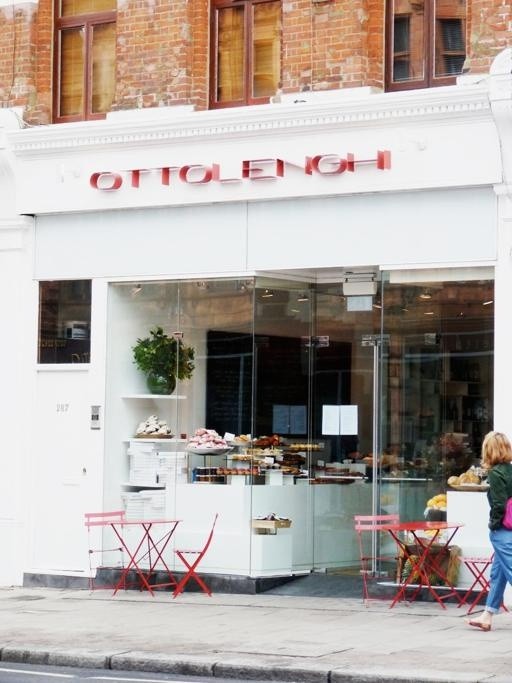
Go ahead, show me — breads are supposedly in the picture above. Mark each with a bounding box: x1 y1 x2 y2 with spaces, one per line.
187 428 229 449
447 468 481 485
280 464 301 475
136 414 171 435
252 435 280 446
235 434 248 442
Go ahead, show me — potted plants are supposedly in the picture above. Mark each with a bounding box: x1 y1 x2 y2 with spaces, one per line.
133 325 197 395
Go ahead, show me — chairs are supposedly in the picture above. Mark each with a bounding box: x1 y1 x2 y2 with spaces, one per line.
355 513 507 612
80 508 221 598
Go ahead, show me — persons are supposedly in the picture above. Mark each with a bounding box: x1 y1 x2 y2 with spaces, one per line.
465 430 512 632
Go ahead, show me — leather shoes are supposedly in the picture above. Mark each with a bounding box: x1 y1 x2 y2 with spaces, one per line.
464 618 491 631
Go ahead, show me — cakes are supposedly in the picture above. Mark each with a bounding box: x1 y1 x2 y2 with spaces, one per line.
288 443 320 451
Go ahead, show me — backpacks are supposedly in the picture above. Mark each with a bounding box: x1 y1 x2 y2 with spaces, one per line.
502 496 512 529
